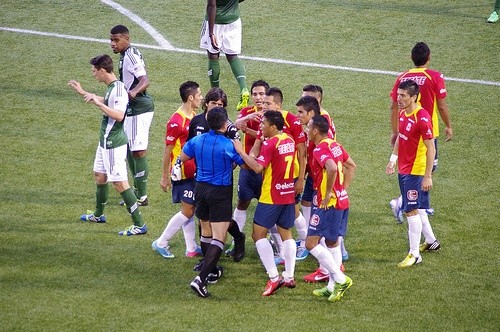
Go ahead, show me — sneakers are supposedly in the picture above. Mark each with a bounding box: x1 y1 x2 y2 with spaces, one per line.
233 233 245 262
420 240 441 252
304 268 329 283
80 212 107 223
313 276 353 303
262 277 296 296
193 259 204 271
397 252 422 267
151 241 174 259
119 223 148 235
208 266 223 283
237 88 250 111
119 195 148 206
189 275 210 298
186 246 203 257
225 244 233 256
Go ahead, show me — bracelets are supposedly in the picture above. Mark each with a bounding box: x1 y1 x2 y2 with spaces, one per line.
390 154 398 162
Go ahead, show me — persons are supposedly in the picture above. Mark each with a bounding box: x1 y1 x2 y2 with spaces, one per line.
231 110 299 296
303 116 353 302
105 25 154 207
293 85 349 261
180 106 262 298
294 95 346 282
487 0 500 23
149 81 205 259
202 0 250 110
225 79 284 257
385 80 442 267
67 54 147 236
386 41 453 223
186 86 246 270
240 88 310 265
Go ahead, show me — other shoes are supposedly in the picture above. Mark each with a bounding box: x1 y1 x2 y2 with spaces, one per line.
296 247 309 260
342 250 349 261
389 198 404 224
486 11 499 22
426 208 435 215
274 256 284 264
